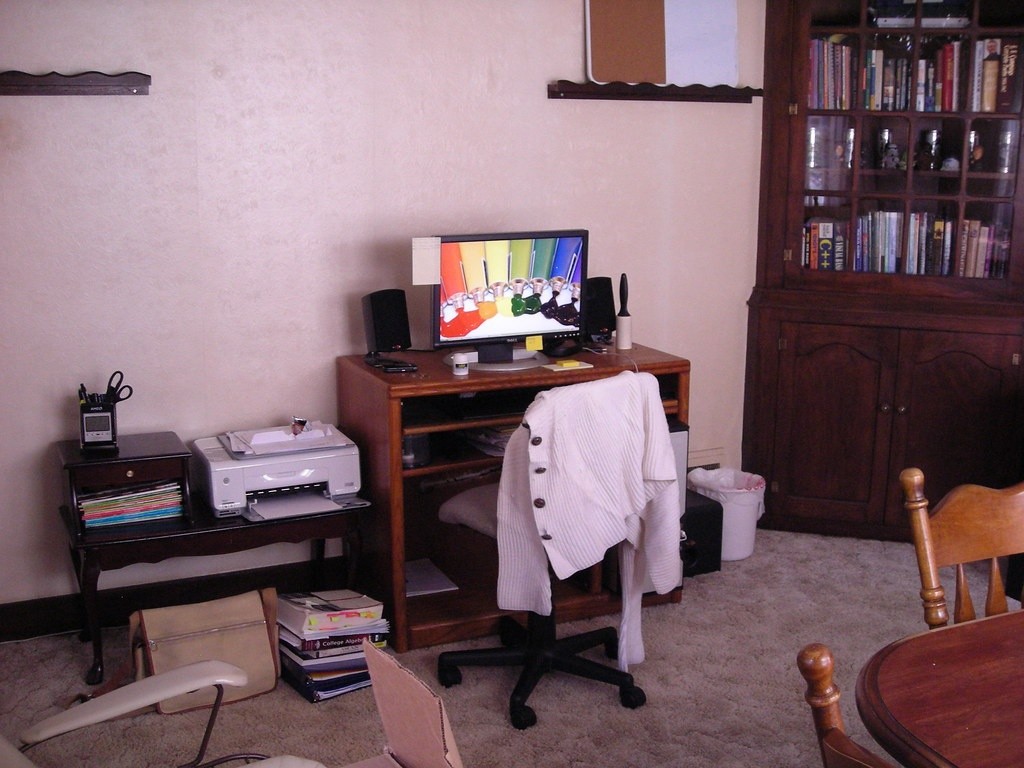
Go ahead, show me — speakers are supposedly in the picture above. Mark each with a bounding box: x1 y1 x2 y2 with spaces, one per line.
585 276 616 349
360 288 412 367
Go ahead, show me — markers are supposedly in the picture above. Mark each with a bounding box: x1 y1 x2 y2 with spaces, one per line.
78 383 90 405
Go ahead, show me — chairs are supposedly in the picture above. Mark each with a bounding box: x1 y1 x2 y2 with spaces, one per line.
432 371 678 729
798 638 911 768
899 467 1024 624
0 658 327 768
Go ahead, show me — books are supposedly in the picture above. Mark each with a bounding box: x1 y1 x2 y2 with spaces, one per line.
279 619 390 703
802 211 1008 278
75 480 183 527
808 38 1018 114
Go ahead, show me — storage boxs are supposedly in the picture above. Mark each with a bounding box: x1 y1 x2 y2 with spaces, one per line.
277 590 382 634
341 635 466 768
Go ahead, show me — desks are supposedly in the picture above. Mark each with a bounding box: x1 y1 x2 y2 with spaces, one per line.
335 340 700 652
68 501 387 686
854 610 1023 767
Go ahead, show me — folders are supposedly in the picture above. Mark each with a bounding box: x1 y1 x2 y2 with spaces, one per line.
280 651 373 703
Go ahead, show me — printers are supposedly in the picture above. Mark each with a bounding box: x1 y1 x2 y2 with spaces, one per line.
193 422 371 522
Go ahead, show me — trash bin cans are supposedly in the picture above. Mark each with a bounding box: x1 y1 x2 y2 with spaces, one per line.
688 468 765 562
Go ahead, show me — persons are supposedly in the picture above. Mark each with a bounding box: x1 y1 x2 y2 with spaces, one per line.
984 41 999 60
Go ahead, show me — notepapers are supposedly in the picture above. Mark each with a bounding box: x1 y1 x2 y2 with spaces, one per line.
556 359 579 369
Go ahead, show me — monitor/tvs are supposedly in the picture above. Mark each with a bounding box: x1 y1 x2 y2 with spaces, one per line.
430 229 588 370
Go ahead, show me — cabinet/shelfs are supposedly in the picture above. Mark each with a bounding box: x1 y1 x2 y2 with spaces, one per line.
734 0 1024 540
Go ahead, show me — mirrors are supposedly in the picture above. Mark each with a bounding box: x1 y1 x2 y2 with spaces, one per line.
584 0 739 88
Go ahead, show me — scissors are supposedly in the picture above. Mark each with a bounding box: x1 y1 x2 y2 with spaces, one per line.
105 371 134 404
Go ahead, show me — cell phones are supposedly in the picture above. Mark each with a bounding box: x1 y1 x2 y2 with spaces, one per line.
384 364 418 373
